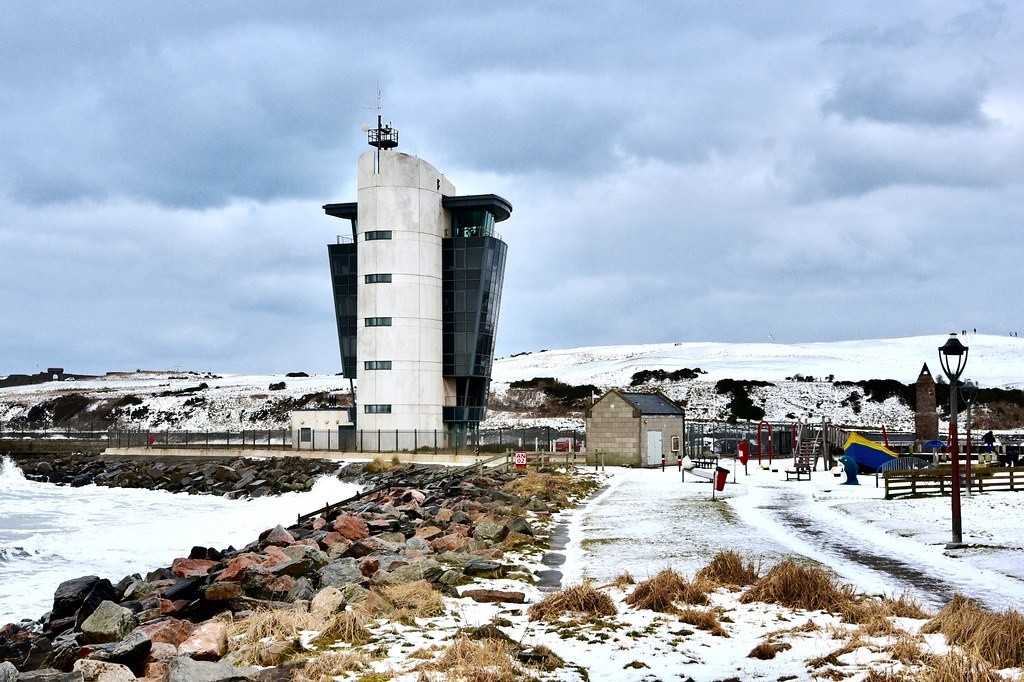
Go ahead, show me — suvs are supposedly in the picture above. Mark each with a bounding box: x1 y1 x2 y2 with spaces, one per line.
717 439 757 455
685 437 722 453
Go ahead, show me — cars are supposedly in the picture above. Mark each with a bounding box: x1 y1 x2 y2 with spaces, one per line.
551 438 581 452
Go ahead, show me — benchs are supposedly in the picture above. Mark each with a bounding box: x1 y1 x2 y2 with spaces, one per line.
875 456 940 486
691 456 718 468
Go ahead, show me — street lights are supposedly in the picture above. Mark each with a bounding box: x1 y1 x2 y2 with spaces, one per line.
937 333 969 541
